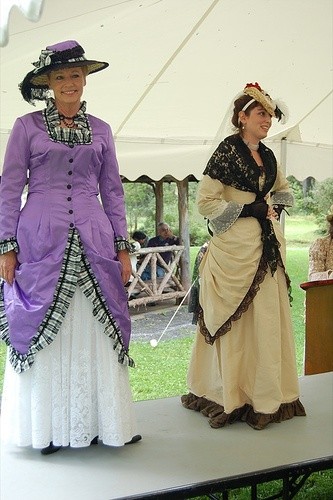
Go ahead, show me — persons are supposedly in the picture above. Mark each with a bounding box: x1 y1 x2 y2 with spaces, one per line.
0 40 142 454
181 83 306 431
308 204 333 281
136 223 179 281
129 231 150 249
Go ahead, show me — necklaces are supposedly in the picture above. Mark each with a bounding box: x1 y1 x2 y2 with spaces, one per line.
59 115 75 128
242 137 259 151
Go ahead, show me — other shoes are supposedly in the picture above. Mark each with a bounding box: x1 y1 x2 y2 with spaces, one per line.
40 441 60 455
124 434 141 444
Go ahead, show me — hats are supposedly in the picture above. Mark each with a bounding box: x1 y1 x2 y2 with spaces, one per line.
243 82 288 125
18 40 109 106
